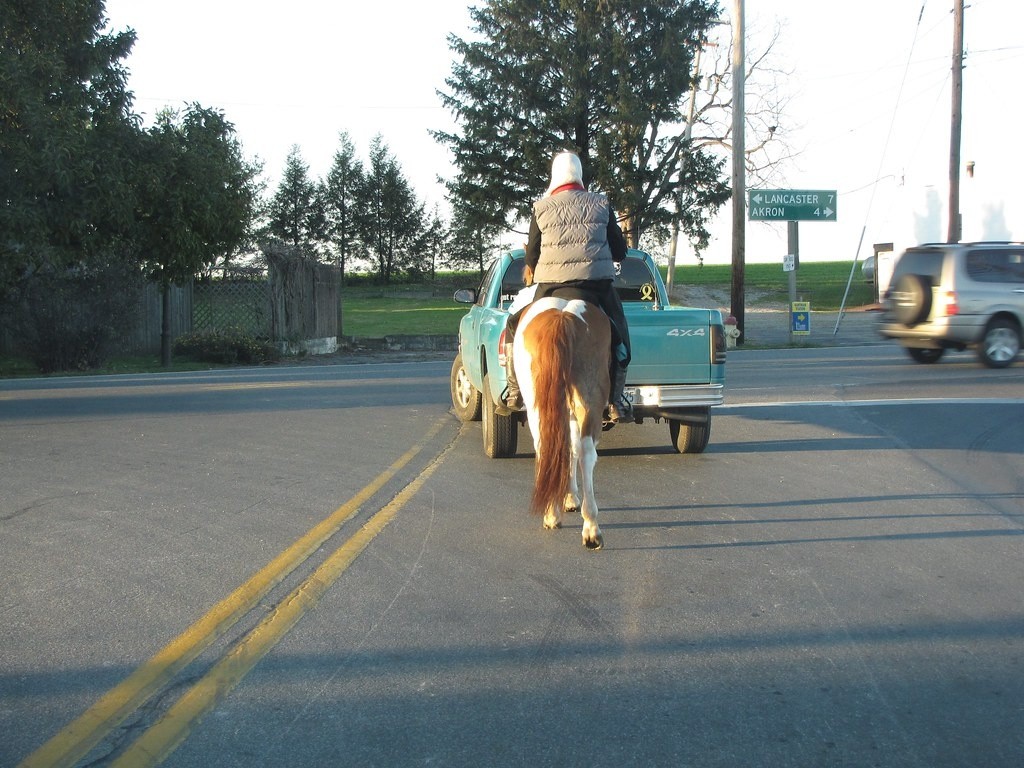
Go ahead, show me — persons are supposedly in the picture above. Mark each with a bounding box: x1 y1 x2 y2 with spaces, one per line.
506 153 634 423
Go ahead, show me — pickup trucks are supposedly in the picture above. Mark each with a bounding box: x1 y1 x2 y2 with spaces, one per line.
450 249 727 459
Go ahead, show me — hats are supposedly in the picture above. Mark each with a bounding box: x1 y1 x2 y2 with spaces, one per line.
542 148 585 197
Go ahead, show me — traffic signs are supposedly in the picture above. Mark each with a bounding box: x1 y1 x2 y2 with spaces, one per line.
792 302 811 336
748 189 837 222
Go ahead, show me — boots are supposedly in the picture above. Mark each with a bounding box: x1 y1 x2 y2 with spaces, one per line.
495 343 523 417
602 368 626 431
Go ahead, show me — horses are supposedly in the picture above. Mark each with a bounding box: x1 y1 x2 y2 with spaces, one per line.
510 296 613 552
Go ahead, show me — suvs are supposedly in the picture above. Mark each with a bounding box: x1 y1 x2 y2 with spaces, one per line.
875 243 1023 370
